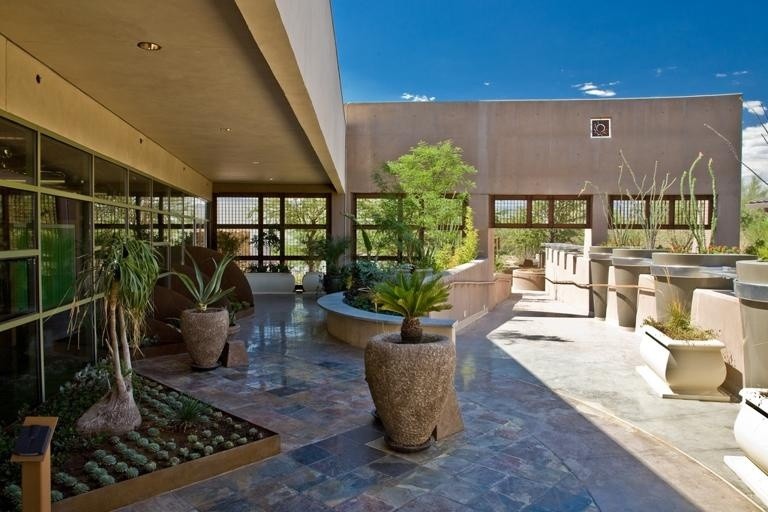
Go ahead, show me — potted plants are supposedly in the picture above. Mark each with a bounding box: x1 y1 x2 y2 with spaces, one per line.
365 267 455 453
248 262 288 273
635 264 731 403
169 248 239 371
298 230 364 292
